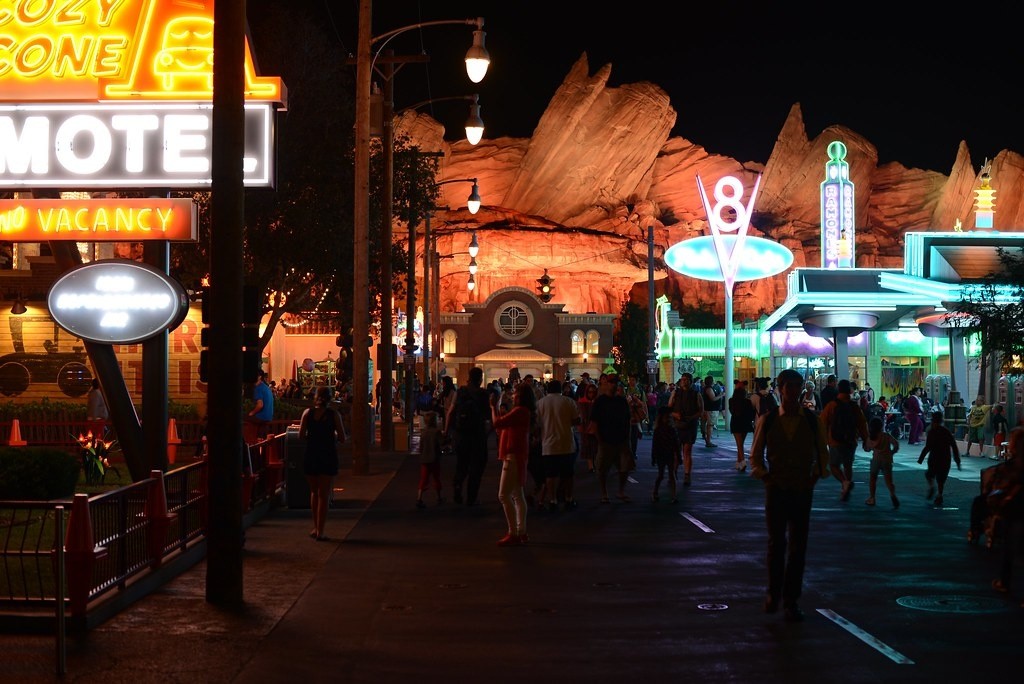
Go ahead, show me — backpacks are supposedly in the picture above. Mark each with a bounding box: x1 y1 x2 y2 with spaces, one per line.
830 407 861 447
758 393 777 417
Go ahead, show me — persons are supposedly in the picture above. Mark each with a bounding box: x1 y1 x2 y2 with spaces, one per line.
372 375 418 421
647 365 726 500
418 359 645 548
295 387 349 544
239 368 275 424
728 360 898 627
277 377 348 401
86 377 109 423
900 384 1024 624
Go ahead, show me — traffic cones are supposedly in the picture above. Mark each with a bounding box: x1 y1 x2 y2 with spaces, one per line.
8 418 28 448
167 418 185 445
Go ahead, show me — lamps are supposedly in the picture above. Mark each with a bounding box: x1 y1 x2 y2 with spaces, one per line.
464 94 485 145
469 257 477 275
468 178 481 214
468 274 475 290
464 17 491 83
469 230 479 257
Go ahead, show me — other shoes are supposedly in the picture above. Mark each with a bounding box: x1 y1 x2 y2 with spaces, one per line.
908 438 927 445
498 532 531 548
897 433 904 441
961 453 1008 460
928 487 944 502
766 591 806 623
407 464 693 507
704 440 901 508
309 530 330 542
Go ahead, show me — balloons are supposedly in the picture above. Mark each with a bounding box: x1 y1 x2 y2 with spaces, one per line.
302 358 315 371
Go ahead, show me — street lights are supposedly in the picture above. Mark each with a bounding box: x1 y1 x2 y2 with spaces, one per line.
354 2 492 477
